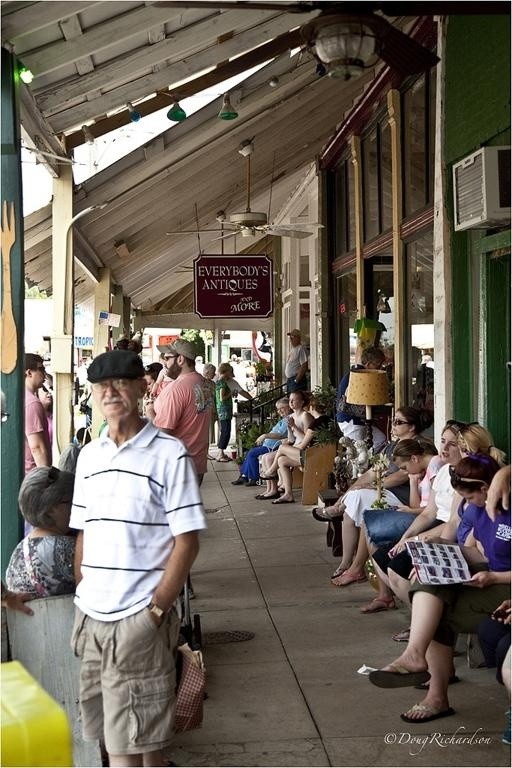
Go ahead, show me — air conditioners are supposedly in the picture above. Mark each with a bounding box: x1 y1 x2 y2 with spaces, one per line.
451 145 510 235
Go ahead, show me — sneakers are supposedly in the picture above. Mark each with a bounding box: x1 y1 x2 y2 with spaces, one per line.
207 454 233 462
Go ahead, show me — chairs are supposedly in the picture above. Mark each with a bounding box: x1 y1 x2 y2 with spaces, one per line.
5 594 110 767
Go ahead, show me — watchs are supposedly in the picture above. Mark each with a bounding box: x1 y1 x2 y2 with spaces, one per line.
147 602 167 621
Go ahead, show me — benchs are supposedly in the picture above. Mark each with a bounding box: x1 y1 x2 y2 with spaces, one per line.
294 440 340 505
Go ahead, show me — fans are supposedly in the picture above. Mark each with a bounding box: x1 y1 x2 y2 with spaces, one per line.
173 211 227 273
164 147 325 242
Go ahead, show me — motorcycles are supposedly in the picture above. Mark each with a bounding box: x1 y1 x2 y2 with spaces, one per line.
243 361 257 392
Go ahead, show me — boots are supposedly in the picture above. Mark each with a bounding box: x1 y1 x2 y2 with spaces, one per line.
231 476 258 487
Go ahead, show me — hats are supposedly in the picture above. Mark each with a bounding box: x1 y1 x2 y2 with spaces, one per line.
287 328 302 336
156 339 196 360
87 350 145 383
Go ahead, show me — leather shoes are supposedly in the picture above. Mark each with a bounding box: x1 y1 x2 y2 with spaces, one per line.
259 471 279 481
255 492 280 500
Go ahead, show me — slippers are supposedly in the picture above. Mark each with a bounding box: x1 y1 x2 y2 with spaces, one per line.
370 663 431 688
400 703 455 723
272 498 295 504
313 507 343 522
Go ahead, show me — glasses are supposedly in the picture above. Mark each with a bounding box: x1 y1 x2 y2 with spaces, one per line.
44 466 61 489
42 385 53 392
92 379 130 391
163 355 177 361
35 367 45 372
395 421 409 425
447 465 489 490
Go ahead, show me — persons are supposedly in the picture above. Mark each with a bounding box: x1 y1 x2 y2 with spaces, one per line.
2 580 37 616
5 463 81 600
19 347 226 534
145 337 213 493
67 347 211 765
226 327 511 753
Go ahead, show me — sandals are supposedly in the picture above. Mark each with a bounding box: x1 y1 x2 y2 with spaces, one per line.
332 571 368 587
360 598 399 615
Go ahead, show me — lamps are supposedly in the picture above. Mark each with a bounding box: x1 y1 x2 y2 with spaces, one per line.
314 0 378 80
345 367 395 465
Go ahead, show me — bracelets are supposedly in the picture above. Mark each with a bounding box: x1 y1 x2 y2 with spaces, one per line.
145 400 154 406
1 588 13 601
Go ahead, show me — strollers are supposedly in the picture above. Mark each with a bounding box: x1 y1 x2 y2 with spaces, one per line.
173 571 205 648
